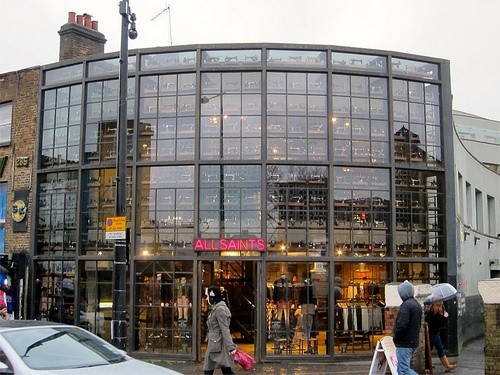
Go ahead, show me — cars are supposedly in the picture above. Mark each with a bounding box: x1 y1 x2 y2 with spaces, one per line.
0 319 185 375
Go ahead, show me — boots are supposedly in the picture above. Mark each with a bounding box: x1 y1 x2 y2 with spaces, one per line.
425 369 431 375
440 356 457 372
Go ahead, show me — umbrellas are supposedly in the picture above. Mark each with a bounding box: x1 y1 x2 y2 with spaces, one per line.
423 283 460 312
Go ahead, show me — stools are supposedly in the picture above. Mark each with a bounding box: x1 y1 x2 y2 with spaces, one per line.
274 338 293 355
299 337 318 355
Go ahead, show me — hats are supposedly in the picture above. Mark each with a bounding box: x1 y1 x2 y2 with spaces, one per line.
206 286 222 305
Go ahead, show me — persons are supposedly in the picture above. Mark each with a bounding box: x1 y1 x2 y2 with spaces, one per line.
272 273 293 339
334 277 343 303
393 281 422 375
152 275 163 323
174 277 192 326
424 302 457 372
202 286 237 375
0 306 11 320
297 279 317 340
220 286 230 309
